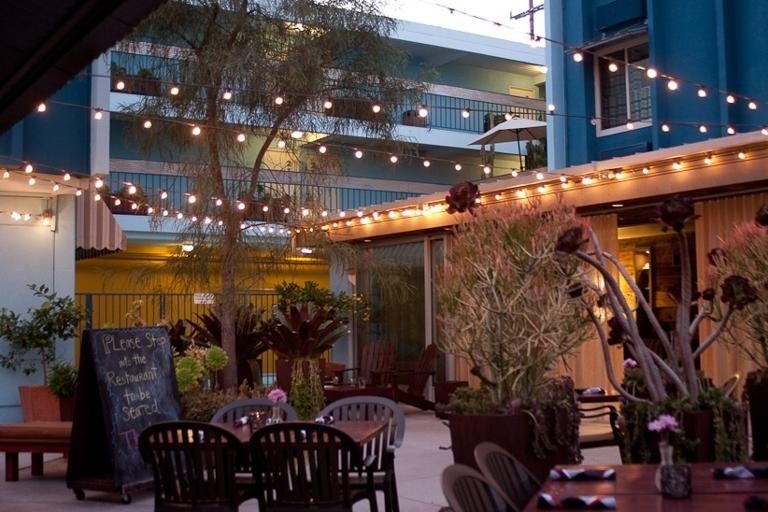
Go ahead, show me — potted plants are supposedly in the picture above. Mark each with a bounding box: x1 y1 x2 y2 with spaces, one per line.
0 282 90 422
438 193 586 472
182 300 350 396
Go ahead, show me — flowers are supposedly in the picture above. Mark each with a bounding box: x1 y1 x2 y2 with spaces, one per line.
648 413 679 443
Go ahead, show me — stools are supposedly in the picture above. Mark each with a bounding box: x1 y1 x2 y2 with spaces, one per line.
0 420 77 481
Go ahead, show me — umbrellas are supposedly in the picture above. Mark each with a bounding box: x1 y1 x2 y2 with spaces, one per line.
467 115 547 173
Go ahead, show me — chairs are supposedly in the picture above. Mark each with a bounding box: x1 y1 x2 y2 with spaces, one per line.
335 334 437 411
440 443 539 512
136 395 407 512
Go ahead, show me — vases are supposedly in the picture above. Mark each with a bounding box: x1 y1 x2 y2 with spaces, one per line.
654 442 675 493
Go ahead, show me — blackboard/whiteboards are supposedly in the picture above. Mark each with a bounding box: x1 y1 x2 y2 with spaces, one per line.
90 325 187 488
